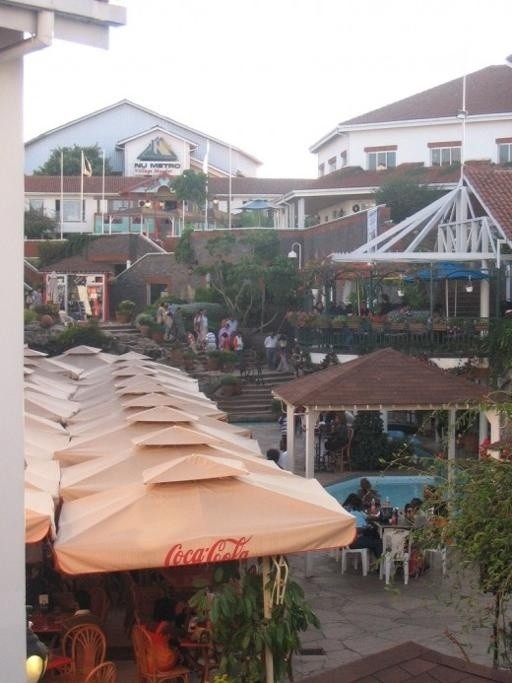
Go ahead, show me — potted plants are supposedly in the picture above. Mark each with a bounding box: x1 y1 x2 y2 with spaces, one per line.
116 300 241 395
290 310 491 332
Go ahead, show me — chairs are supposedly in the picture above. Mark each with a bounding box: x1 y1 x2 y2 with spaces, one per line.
341 528 447 584
43 585 190 683
331 427 354 473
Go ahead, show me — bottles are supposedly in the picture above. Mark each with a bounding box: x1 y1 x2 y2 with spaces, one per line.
392 508 398 525
370 497 376 514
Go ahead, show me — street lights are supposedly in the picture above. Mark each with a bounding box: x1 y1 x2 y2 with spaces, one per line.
288 242 302 269
456 109 468 186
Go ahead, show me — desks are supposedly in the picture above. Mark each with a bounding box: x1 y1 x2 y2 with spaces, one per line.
373 517 416 543
28 611 63 647
179 637 229 683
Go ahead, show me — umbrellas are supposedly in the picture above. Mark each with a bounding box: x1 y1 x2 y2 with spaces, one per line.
442 268 488 318
404 263 462 320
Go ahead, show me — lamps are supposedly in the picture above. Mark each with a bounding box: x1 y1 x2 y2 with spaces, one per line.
26 653 49 683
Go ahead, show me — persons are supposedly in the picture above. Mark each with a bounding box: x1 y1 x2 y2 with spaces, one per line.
337 474 449 579
146 597 206 679
265 446 281 468
278 435 288 469
159 301 290 370
358 293 400 319
325 413 350 463
